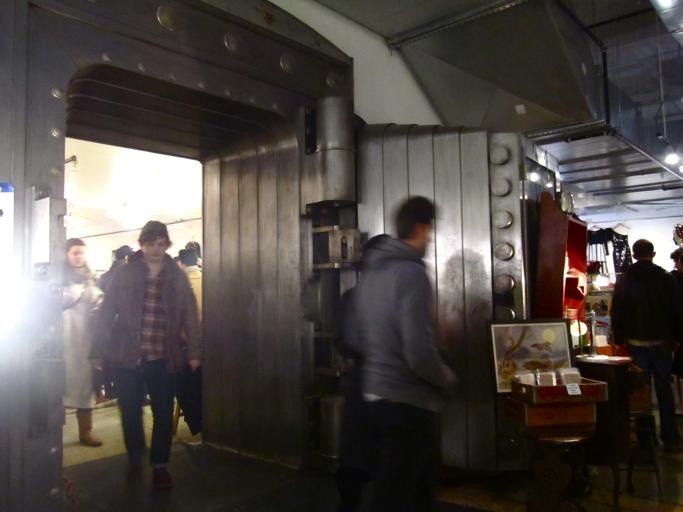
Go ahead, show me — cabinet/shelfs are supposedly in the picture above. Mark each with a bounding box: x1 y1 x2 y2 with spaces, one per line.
536 191 589 322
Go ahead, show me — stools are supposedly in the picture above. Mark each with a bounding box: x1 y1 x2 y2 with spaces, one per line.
521 426 596 512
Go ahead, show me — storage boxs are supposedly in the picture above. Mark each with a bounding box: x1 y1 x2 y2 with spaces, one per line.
502 377 609 429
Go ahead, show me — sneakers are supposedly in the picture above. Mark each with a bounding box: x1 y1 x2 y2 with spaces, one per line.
185 434 202 445
80 427 102 446
154 468 171 489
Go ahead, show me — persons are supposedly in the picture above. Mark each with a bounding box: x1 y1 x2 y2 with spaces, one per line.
63 220 202 487
670 247 683 379
609 240 683 454
334 234 391 512
346 196 457 512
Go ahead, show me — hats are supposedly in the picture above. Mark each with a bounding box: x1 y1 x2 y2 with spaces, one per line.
141 221 167 234
